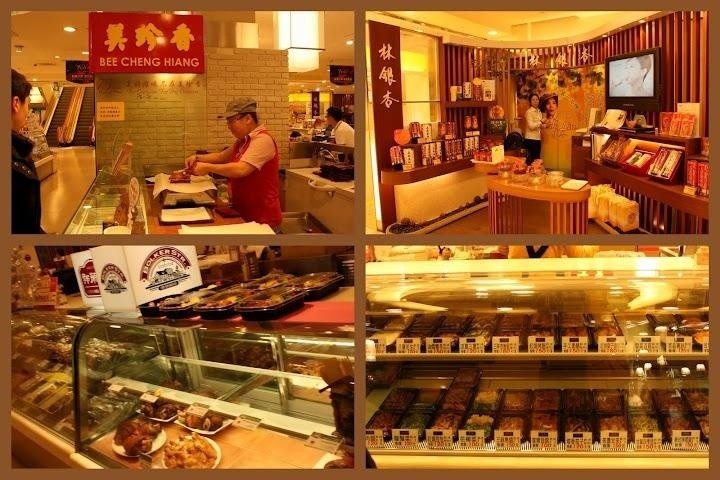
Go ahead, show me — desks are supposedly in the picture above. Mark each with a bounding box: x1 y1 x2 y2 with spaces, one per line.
484 169 587 233
65 179 256 235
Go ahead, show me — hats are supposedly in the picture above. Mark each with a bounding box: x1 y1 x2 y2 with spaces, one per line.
216 97 257 119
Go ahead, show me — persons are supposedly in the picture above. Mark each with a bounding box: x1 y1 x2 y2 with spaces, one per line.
539 92 559 119
11 68 48 234
326 106 354 148
183 96 283 236
610 55 652 97
524 93 548 164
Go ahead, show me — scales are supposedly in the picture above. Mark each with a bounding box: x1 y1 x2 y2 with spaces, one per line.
159 190 216 209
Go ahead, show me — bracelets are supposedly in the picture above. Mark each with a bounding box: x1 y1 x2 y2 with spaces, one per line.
195 155 197 161
540 120 544 124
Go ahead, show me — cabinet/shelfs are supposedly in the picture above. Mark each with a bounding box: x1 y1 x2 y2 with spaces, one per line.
12 305 355 468
362 247 714 470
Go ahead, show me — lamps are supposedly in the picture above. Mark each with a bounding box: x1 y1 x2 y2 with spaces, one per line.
253 8 327 73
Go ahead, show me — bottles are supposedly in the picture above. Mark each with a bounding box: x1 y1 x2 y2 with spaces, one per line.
496 159 564 188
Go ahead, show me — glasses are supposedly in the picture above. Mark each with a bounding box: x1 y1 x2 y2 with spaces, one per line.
227 113 248 124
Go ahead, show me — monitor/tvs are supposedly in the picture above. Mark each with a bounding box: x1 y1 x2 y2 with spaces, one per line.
605 47 661 112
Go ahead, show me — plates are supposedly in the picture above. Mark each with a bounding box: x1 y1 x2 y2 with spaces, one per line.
111 407 235 469
